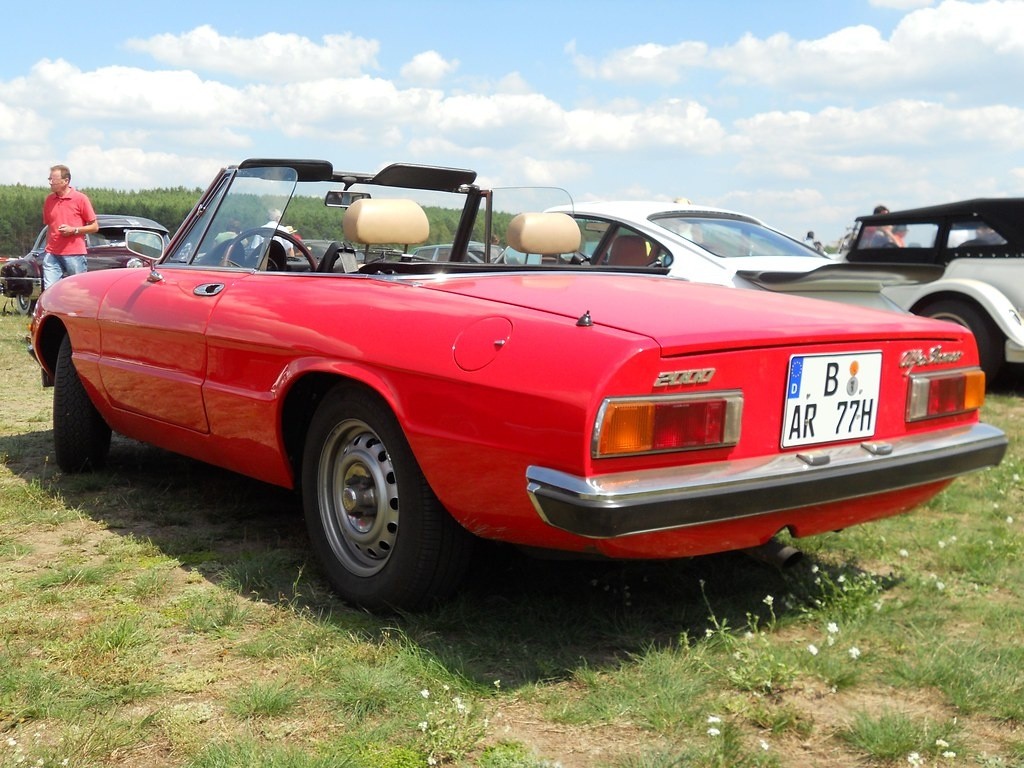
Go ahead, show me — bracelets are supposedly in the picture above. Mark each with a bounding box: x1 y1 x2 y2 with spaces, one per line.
75 227 79 235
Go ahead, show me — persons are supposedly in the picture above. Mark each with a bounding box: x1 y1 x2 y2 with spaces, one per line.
42 165 99 291
491 234 499 245
859 205 1007 247
804 231 828 257
246 209 295 258
284 226 304 257
212 220 249 248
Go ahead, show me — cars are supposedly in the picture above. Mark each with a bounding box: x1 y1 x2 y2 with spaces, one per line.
27 158 1009 618
0 215 172 316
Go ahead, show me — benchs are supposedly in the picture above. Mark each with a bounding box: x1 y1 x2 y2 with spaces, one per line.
344 188 672 278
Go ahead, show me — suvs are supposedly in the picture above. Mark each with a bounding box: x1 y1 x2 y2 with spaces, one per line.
825 198 1023 391
